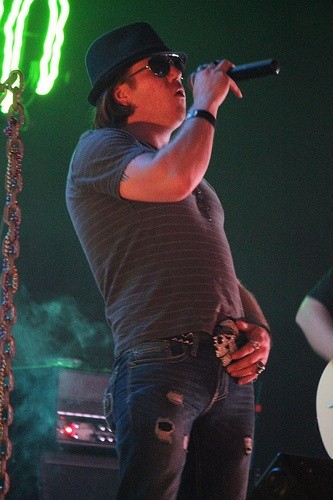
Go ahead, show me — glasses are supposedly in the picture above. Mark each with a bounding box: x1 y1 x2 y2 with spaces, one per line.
127 52 187 78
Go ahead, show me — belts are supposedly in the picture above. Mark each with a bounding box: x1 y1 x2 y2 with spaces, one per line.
161 316 249 367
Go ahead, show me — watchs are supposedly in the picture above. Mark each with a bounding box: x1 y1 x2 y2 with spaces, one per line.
187 109 217 126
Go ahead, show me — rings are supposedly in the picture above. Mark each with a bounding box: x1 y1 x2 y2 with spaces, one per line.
249 340 261 350
255 361 265 377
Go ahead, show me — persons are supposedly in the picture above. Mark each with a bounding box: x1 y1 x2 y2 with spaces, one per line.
295 265 333 363
65 21 272 500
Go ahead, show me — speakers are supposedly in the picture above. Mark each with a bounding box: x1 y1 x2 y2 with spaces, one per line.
247 452 333 500
39 450 120 500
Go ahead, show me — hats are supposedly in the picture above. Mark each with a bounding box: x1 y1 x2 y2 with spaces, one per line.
84 22 187 107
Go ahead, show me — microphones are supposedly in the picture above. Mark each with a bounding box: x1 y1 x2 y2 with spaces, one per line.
187 58 280 93
